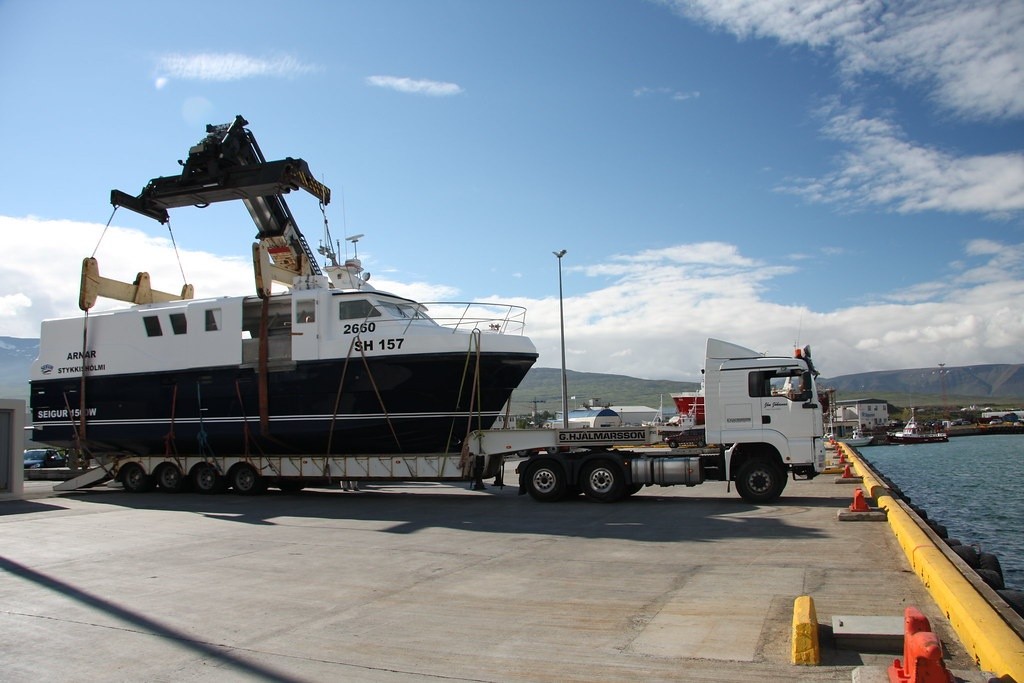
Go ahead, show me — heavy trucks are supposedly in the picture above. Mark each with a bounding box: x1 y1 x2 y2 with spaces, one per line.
23 286 841 501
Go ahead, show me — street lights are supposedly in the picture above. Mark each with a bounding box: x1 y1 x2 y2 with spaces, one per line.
553 249 569 429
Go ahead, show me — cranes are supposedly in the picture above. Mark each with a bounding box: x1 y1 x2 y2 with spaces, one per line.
108 115 332 285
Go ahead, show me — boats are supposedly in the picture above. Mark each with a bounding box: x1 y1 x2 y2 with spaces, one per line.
889 407 951 444
26 287 539 454
